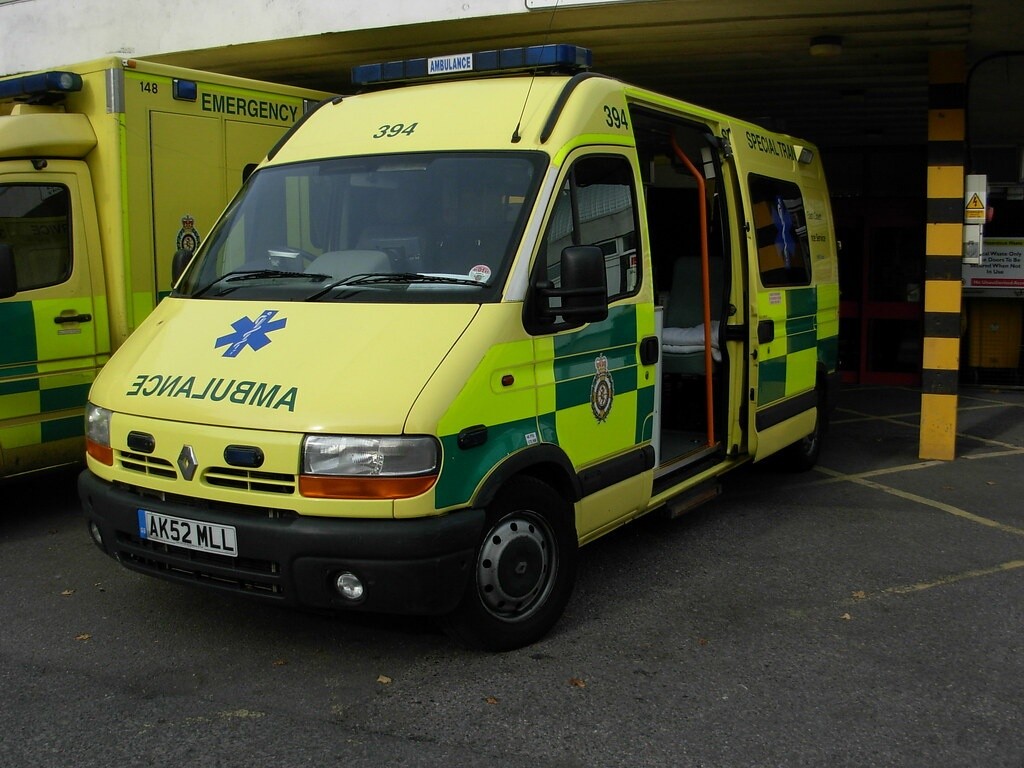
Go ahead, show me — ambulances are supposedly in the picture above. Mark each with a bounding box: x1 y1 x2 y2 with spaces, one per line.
77 40 848 652
0 56 364 485
